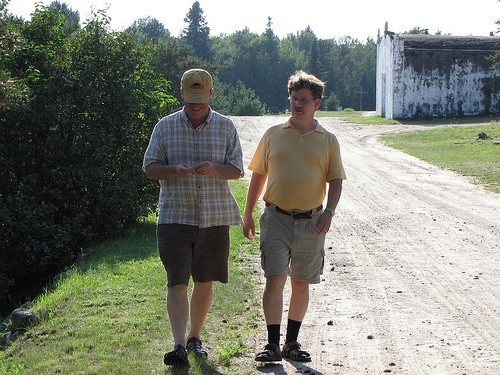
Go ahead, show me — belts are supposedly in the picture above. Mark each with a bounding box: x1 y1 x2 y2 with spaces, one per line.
265 201 323 220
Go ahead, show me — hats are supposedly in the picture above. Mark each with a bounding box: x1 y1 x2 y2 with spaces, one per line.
181 69 212 103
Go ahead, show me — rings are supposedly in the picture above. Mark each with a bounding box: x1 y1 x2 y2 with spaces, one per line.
324 229 327 232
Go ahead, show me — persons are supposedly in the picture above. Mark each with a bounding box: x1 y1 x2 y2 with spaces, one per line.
141 68 244 366
242 70 347 361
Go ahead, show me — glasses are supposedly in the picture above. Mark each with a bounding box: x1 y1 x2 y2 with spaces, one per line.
291 96 315 104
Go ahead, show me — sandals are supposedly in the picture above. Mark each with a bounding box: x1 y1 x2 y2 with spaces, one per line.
282 342 311 362
164 346 189 366
254 343 282 362
187 337 208 359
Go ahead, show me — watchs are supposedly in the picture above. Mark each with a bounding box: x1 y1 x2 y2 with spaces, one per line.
325 207 335 216
